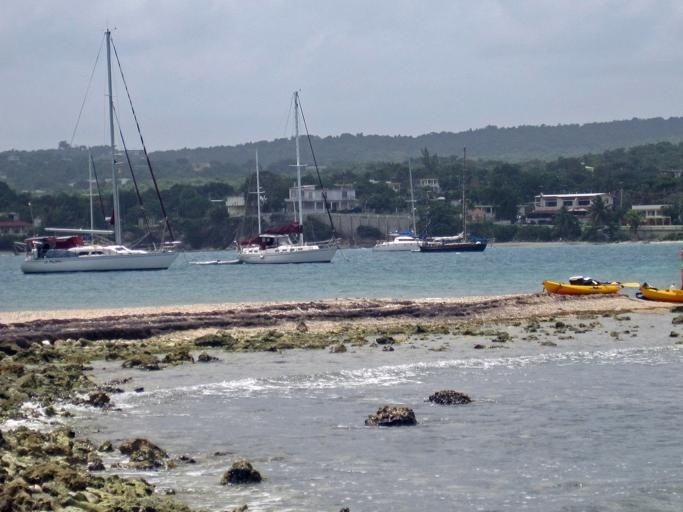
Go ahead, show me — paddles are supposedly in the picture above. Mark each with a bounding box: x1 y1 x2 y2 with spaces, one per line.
601 282 640 287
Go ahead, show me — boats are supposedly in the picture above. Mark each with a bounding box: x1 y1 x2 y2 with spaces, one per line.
640 283 683 302
374 147 490 251
544 280 622 294
234 91 342 264
21 30 181 273
